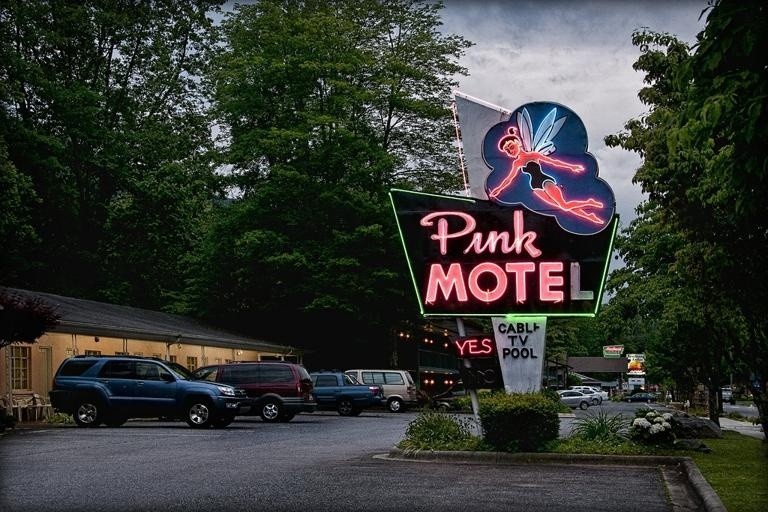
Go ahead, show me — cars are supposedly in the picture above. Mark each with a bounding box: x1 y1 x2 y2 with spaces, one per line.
592 387 600 391
557 389 592 411
297 373 386 417
666 391 673 399
574 389 602 406
624 393 657 403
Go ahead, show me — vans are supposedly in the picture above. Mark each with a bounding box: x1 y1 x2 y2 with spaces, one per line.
188 359 316 421
343 369 417 415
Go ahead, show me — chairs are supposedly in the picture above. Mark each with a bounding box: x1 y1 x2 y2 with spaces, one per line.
1 394 30 423
33 394 53 420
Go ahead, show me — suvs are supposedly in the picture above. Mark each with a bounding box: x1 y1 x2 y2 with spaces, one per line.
432 381 472 411
720 388 736 405
49 353 242 427
75 353 249 427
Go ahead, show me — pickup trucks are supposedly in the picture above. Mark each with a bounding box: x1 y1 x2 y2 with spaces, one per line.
572 386 609 405
